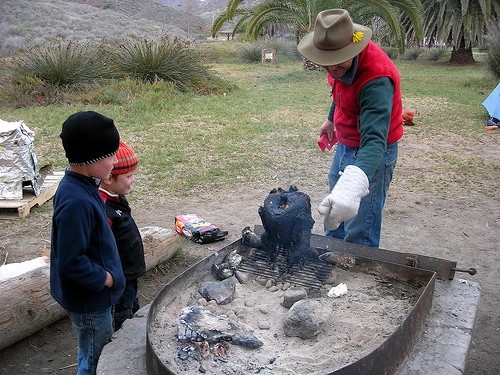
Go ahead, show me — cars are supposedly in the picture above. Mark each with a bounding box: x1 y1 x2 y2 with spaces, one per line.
420 37 446 48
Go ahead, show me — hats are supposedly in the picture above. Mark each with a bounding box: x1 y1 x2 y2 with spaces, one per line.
111 140 138 174
297 9 372 66
59 111 119 165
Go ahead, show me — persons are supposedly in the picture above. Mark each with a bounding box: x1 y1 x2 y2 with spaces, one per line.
294 7 405 250
429 35 435 49
47 110 127 375
96 139 148 331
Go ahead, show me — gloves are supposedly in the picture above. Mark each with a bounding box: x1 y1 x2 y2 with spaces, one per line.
318 165 370 230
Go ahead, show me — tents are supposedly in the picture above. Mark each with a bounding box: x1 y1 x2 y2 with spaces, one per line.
481 82 500 128
